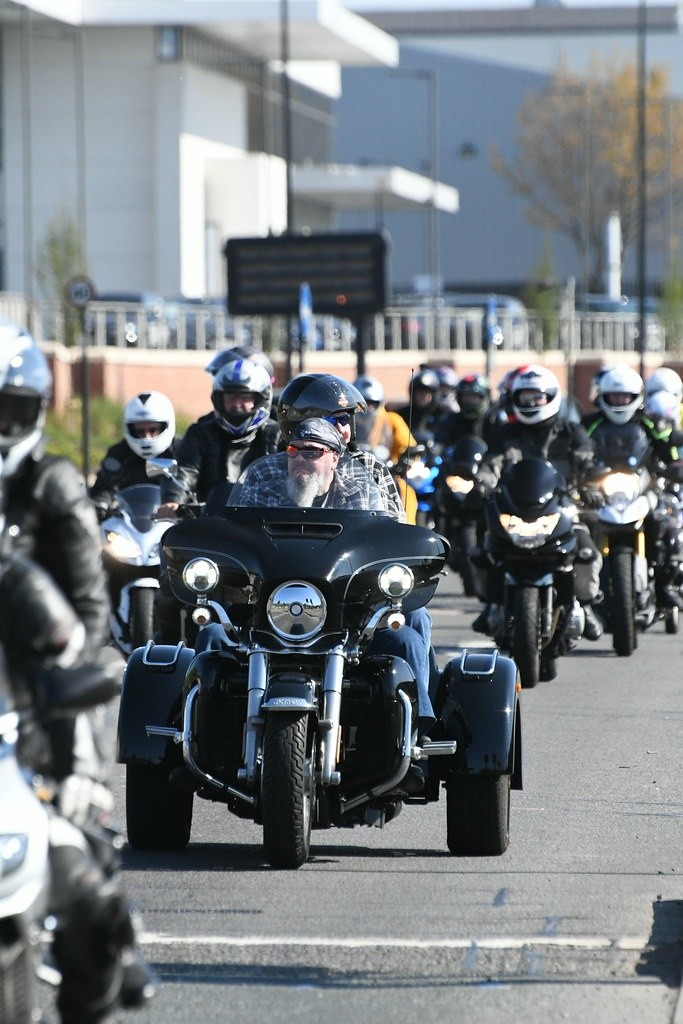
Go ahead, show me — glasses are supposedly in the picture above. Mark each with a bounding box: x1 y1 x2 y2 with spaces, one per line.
286 443 333 460
324 414 351 428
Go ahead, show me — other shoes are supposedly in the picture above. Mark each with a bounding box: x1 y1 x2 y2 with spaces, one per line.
471 604 490 635
49 813 159 1024
579 601 603 639
659 581 683 608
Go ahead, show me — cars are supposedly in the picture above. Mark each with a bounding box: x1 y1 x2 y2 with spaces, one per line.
81 291 356 350
368 295 664 349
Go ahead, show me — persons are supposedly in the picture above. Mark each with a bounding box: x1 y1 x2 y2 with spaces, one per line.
464 365 606 640
349 364 492 529
0 333 162 1024
165 417 438 795
581 365 682 610
92 388 185 523
273 374 434 742
153 346 287 526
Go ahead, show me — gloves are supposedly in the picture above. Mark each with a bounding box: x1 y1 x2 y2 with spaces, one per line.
580 479 612 511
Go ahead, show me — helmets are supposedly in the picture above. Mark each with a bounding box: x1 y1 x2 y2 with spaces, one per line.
455 373 490 419
1 321 53 476
123 390 176 459
204 345 274 377
435 366 457 407
409 370 441 413
509 363 561 424
496 371 511 403
644 366 682 431
275 373 368 444
597 367 645 424
210 360 273 437
352 375 383 402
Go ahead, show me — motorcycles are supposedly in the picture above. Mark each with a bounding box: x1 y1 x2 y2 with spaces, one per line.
114 507 524 870
457 435 612 689
346 408 683 656
0 664 120 1023
99 484 182 660
